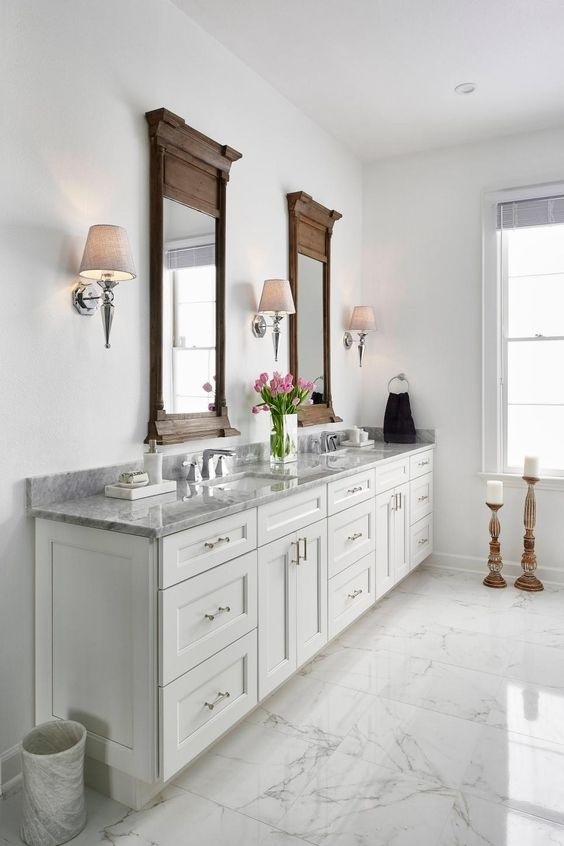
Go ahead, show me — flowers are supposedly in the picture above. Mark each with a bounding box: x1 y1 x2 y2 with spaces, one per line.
201 375 215 410
249 370 316 457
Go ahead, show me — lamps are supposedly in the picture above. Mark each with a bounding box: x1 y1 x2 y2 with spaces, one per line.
343 304 377 367
71 224 137 350
252 280 296 360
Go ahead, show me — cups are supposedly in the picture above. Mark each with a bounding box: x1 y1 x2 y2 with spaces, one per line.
335 431 346 445
309 439 322 454
360 432 369 442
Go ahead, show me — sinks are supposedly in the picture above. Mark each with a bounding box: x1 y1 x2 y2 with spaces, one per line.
320 447 394 457
194 470 298 494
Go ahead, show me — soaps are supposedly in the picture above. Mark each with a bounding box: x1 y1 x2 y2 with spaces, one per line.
121 471 147 483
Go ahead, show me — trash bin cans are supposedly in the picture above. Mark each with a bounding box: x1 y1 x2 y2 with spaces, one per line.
19 719 88 846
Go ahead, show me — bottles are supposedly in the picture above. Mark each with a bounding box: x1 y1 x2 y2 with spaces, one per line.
348 425 360 443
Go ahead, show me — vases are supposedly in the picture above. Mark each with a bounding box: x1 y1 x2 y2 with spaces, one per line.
269 413 299 462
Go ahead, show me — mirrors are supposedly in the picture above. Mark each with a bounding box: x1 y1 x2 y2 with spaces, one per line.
144 108 241 445
286 189 344 429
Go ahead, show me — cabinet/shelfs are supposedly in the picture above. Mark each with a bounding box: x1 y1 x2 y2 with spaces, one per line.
159 506 260 782
409 449 435 572
376 456 411 600
326 467 377 643
258 483 329 701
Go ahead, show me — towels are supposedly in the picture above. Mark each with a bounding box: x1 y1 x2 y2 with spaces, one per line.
382 392 417 443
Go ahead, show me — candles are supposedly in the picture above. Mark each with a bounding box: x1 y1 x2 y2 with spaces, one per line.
486 480 503 504
523 454 540 477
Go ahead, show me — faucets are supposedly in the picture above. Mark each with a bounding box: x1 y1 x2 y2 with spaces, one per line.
320 431 337 453
202 448 236 478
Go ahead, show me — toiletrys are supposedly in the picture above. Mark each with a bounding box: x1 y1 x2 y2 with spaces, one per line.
350 425 360 442
143 439 164 483
